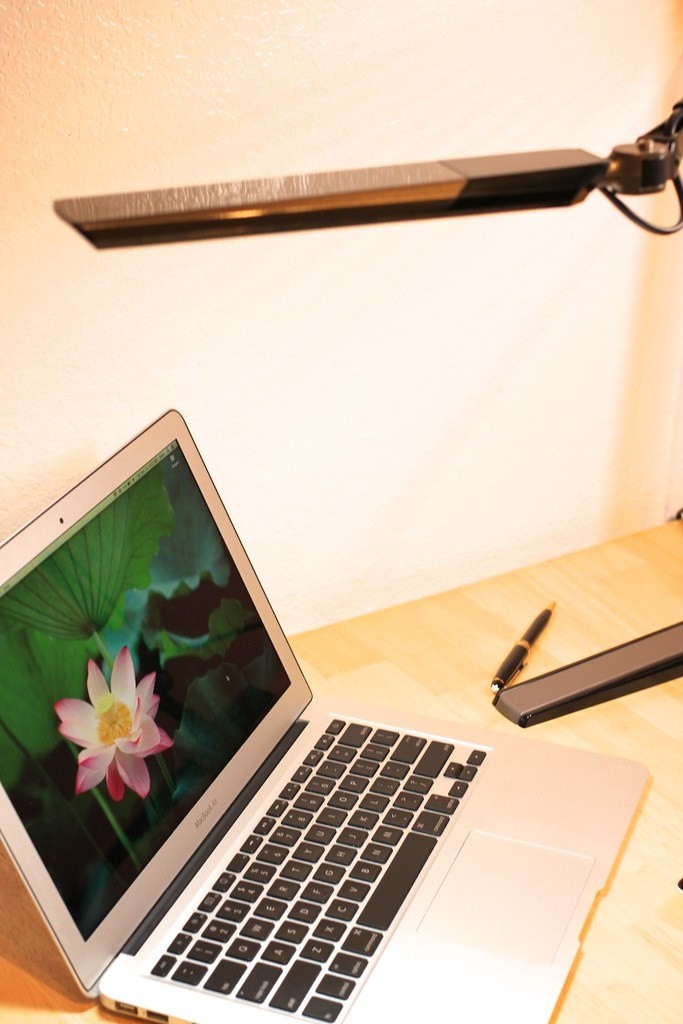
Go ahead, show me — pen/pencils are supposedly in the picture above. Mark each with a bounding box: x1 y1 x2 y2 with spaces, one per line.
489 599 559 694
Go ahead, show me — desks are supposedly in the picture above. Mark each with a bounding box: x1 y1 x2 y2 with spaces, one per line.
0 518 682 1024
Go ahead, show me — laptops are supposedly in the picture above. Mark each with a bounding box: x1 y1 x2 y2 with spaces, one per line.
0 407 648 1024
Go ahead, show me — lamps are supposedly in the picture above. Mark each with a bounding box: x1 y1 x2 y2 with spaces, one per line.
50 92 682 729
